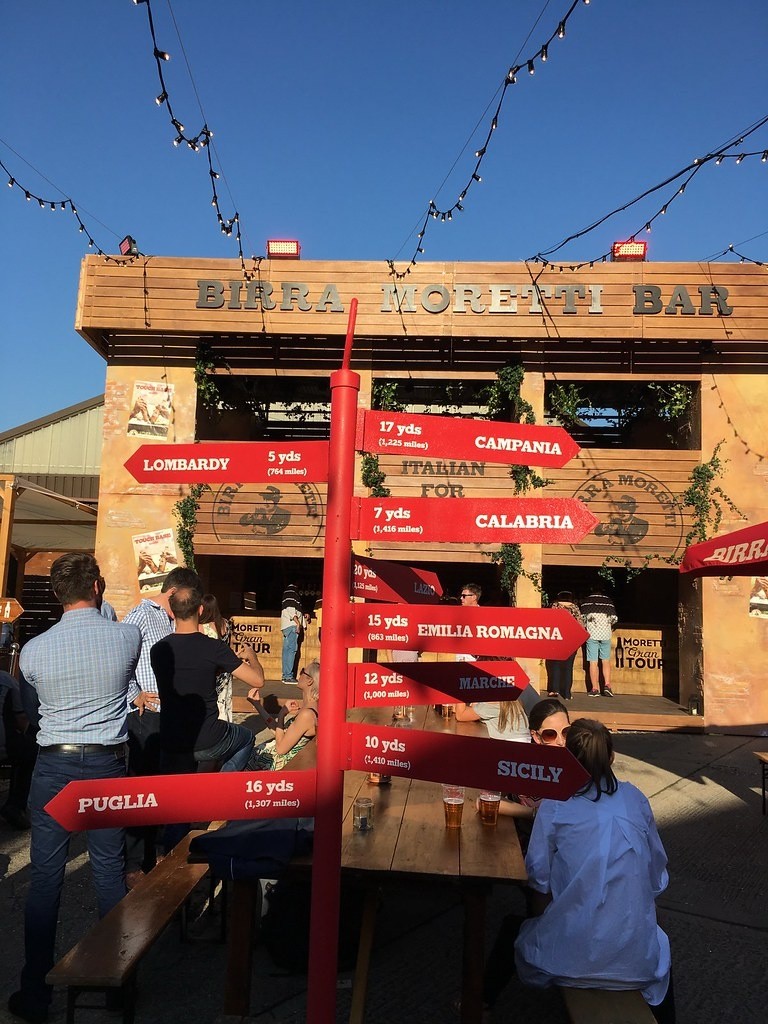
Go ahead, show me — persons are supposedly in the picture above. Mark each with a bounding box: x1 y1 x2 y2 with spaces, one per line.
246 657 320 772
456 655 542 745
7 553 144 1024
456 584 482 663
121 568 206 890
477 698 573 860
151 586 266 855
391 648 423 662
582 612 621 698
280 573 311 685
0 668 43 831
545 591 581 702
449 719 675 1024
313 608 323 642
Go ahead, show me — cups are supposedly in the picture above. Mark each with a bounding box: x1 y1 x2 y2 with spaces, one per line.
480 789 502 826
434 703 456 717
440 783 467 831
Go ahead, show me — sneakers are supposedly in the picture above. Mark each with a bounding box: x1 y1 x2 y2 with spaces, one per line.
586 689 600 697
604 687 614 697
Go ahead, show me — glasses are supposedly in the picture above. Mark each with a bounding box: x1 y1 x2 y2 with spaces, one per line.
460 594 475 599
300 668 314 679
535 726 572 742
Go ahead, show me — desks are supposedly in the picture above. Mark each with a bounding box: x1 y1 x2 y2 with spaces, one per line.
187 703 529 1024
752 752 768 815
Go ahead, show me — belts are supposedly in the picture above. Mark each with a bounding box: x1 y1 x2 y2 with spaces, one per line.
38 742 126 753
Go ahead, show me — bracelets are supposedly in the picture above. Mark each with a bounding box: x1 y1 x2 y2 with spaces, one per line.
533 806 535 817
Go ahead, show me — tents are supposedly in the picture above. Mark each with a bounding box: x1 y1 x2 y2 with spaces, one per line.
680 521 768 575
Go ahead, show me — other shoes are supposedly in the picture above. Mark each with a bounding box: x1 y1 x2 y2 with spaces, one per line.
565 696 574 700
7 992 49 1024
0 802 31 829
124 869 146 890
106 987 139 1011
547 691 559 696
282 678 301 685
449 999 490 1020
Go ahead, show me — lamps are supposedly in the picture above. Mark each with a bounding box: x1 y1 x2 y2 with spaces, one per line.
119 236 138 255
267 240 302 260
610 241 646 262
688 694 701 715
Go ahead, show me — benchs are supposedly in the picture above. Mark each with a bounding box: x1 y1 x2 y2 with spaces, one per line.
46 830 227 1023
560 988 659 1024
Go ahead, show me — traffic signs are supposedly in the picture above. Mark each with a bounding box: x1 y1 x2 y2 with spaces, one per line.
341 720 594 800
348 601 592 663
42 768 318 833
122 438 329 485
356 408 582 471
350 495 602 546
350 553 444 606
346 658 532 712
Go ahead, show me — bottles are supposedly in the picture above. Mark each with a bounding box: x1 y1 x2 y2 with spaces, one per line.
353 798 375 829
368 705 416 783
